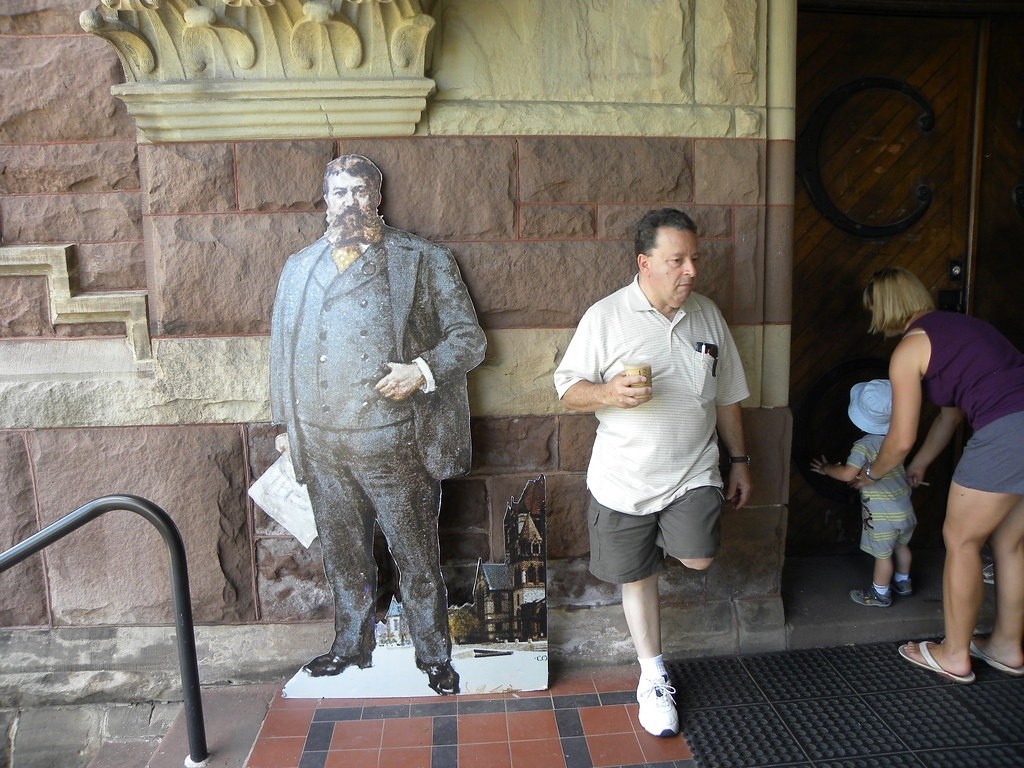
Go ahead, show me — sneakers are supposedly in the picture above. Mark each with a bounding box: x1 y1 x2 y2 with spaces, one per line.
635 674 680 737
982 563 994 585
890 578 913 596
850 585 892 608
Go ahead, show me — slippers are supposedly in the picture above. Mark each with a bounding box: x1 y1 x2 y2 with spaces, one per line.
898 638 1024 684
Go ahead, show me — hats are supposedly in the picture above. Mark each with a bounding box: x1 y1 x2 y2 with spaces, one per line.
847 379 892 435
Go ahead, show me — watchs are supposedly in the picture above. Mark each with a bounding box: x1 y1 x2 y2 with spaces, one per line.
728 454 751 467
864 466 881 482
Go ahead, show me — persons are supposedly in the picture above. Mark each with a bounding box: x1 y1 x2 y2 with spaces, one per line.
845 264 1024 684
809 380 918 608
552 208 755 738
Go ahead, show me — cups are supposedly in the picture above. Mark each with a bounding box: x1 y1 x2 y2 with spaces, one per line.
621 355 652 398
